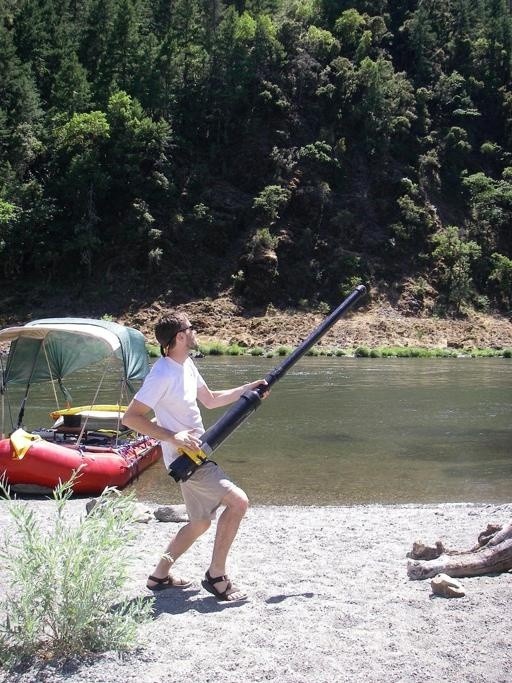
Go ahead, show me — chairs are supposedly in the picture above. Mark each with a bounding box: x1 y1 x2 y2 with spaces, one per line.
53 414 87 441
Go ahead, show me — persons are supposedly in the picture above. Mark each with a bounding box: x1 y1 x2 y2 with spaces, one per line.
121 309 271 601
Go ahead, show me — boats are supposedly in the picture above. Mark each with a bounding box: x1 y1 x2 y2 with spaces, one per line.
0 405 163 496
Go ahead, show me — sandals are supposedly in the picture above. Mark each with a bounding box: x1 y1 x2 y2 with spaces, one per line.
202 570 248 601
146 574 194 590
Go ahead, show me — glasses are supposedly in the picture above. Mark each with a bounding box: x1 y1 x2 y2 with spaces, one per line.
166 322 195 347
